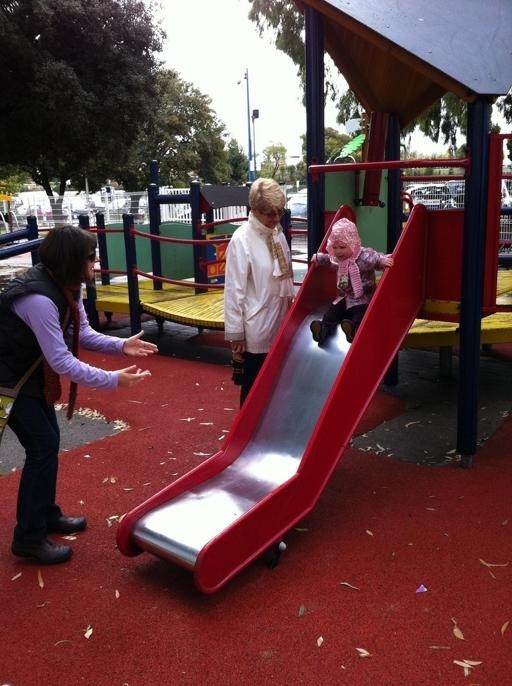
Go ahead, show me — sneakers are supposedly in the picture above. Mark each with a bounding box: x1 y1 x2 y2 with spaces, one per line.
310 320 326 341
341 318 355 343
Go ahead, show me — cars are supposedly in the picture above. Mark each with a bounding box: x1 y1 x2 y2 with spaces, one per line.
446 179 464 208
402 179 455 209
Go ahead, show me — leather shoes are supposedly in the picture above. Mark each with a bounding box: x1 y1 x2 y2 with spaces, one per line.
46 515 85 533
11 538 71 563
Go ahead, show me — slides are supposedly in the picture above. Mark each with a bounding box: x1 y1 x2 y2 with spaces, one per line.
115 202 428 594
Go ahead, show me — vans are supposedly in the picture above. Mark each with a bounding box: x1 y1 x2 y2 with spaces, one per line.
284 187 309 228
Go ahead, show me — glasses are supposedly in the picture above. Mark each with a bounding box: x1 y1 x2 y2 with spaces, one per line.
88 250 96 262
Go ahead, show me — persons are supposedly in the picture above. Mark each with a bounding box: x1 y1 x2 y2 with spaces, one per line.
308 217 395 342
223 175 297 409
0 224 160 563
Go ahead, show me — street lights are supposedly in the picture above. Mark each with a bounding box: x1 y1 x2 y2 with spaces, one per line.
237 67 253 181
